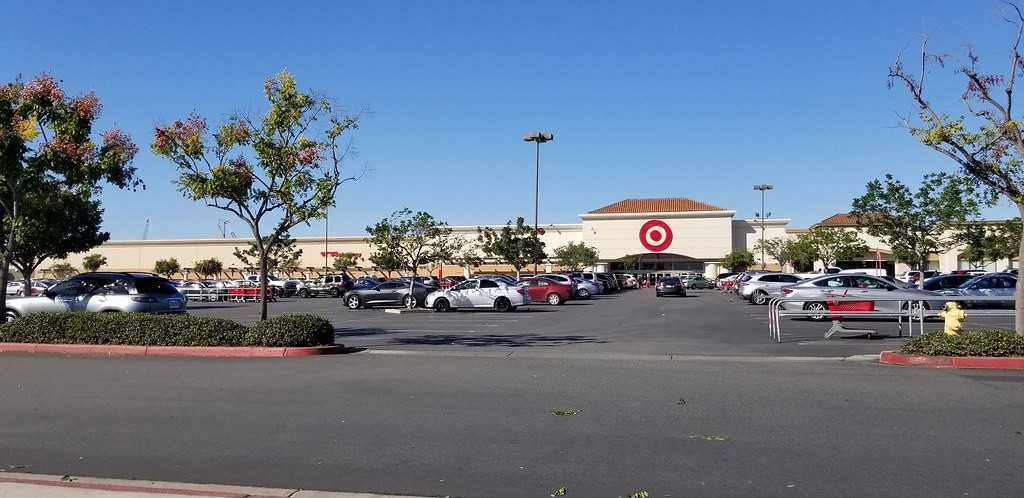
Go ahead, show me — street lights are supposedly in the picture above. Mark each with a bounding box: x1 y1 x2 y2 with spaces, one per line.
523 131 553 276
753 184 773 271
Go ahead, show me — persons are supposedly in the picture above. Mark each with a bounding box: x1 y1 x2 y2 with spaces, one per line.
932 270 939 277
632 275 650 288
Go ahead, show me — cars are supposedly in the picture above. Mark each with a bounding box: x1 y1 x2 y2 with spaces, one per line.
716 270 807 305
782 274 946 322
170 271 639 314
5 277 56 297
656 276 688 297
917 268 1018 315
683 276 709 290
5 272 189 323
877 276 919 289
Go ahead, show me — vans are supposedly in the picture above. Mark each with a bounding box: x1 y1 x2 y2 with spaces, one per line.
900 271 942 282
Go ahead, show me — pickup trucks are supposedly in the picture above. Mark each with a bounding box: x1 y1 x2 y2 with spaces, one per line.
796 266 843 279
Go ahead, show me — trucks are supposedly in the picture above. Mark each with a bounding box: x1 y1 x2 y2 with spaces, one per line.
838 268 888 276
